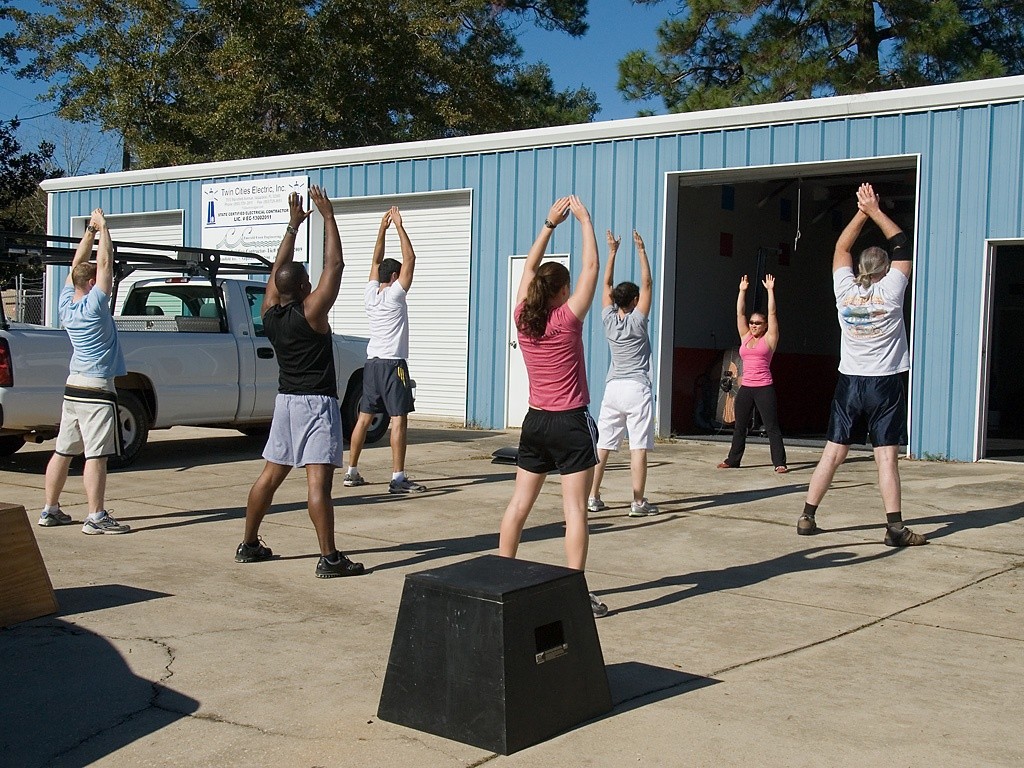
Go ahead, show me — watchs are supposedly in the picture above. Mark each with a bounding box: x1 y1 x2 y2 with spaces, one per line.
87 225 97 233
287 226 298 235
545 219 556 228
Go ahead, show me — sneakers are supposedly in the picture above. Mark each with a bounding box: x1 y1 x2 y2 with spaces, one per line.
38 503 71 527
797 514 820 535
884 524 926 546
589 592 608 618
235 535 272 564
587 491 604 511
344 472 364 487
389 474 426 493
81 508 130 535
628 499 659 516
314 550 365 578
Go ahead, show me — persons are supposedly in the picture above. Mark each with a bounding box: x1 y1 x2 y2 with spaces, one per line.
717 274 787 474
40 209 131 535
798 182 926 547
235 186 363 576
499 195 606 619
588 229 659 517
344 204 427 494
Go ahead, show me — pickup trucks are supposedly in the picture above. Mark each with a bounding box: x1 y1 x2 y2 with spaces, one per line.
0 275 393 475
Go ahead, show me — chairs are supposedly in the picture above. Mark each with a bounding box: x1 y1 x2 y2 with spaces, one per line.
200 304 218 318
143 306 164 316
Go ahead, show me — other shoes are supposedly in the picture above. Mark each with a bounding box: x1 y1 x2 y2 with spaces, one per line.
718 463 729 468
776 465 787 472
